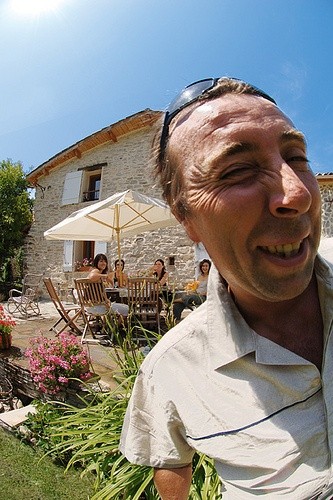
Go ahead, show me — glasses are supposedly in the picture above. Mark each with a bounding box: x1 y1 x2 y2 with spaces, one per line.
159 77 277 172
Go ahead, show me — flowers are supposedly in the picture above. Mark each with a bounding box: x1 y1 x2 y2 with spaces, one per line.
25 332 95 396
0 305 17 335
74 257 93 268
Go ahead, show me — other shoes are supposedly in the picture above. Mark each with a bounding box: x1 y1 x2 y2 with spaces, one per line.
100 326 107 334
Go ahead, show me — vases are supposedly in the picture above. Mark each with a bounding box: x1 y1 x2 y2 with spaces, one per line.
81 372 101 388
75 267 92 272
0 333 13 349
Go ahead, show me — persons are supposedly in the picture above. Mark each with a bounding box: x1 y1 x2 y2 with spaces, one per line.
83 254 129 332
119 76 333 500
112 259 128 288
171 259 211 325
153 259 169 297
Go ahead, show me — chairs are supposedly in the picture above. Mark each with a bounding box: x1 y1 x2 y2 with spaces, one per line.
127 277 161 344
42 276 85 337
7 273 43 319
73 277 122 346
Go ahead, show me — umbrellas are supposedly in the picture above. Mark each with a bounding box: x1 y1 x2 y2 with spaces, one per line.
43 189 180 305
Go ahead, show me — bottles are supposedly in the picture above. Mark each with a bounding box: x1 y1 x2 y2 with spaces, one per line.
113 272 118 288
